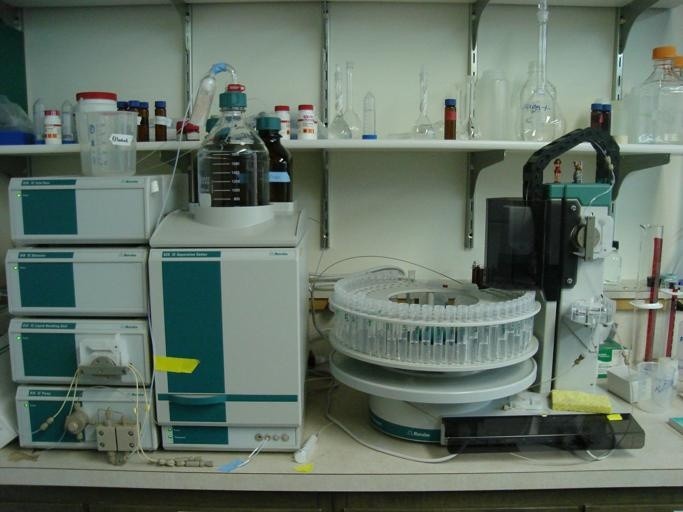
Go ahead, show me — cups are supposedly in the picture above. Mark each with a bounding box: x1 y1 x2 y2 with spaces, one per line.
83 107 136 173
638 361 674 413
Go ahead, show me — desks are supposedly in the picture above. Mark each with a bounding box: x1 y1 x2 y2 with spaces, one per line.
0 359 683 512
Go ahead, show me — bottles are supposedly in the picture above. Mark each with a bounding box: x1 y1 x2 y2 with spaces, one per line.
590 102 612 132
637 44 683 143
197 92 317 206
326 63 375 139
331 269 537 369
32 94 166 147
410 3 566 143
603 247 621 283
